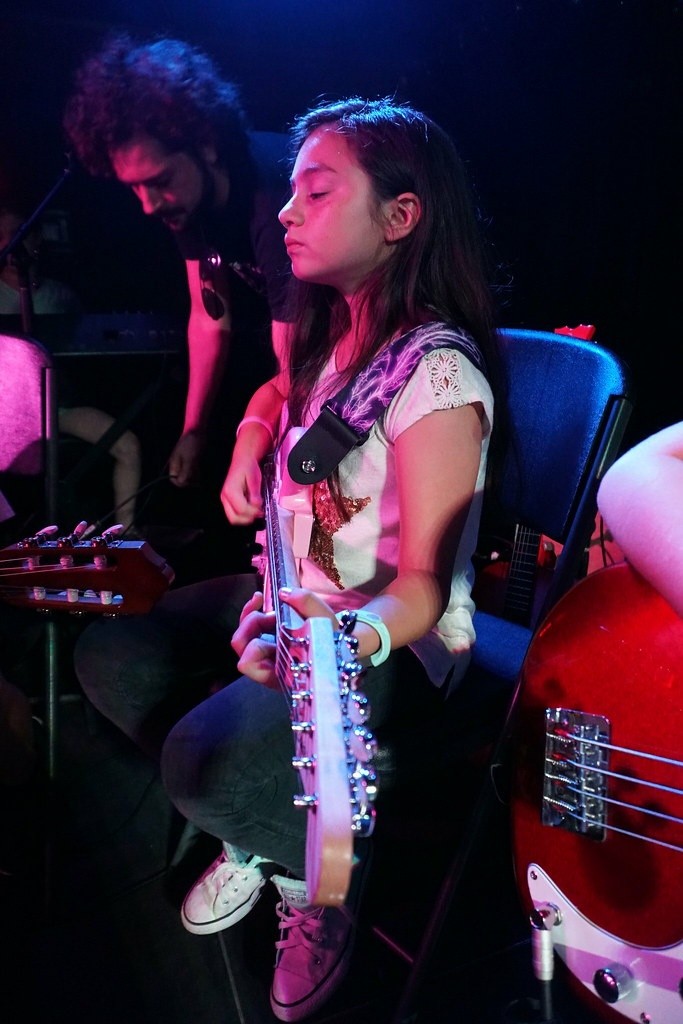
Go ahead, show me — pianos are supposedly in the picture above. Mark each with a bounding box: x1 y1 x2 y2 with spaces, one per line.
0 309 187 357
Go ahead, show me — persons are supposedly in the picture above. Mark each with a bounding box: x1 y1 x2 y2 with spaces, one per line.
63 38 319 487
80 98 510 1022
0 205 144 544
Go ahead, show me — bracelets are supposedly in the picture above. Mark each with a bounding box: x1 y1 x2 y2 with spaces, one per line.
335 609 390 668
235 416 275 441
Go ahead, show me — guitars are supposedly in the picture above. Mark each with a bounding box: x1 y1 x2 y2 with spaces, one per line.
504 323 597 628
0 520 179 620
250 422 379 912
505 558 683 1024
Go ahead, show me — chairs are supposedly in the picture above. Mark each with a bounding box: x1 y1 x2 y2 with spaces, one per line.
200 325 626 1024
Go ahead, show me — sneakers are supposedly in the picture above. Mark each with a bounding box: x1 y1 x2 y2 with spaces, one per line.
180 839 276 936
270 838 374 1021
